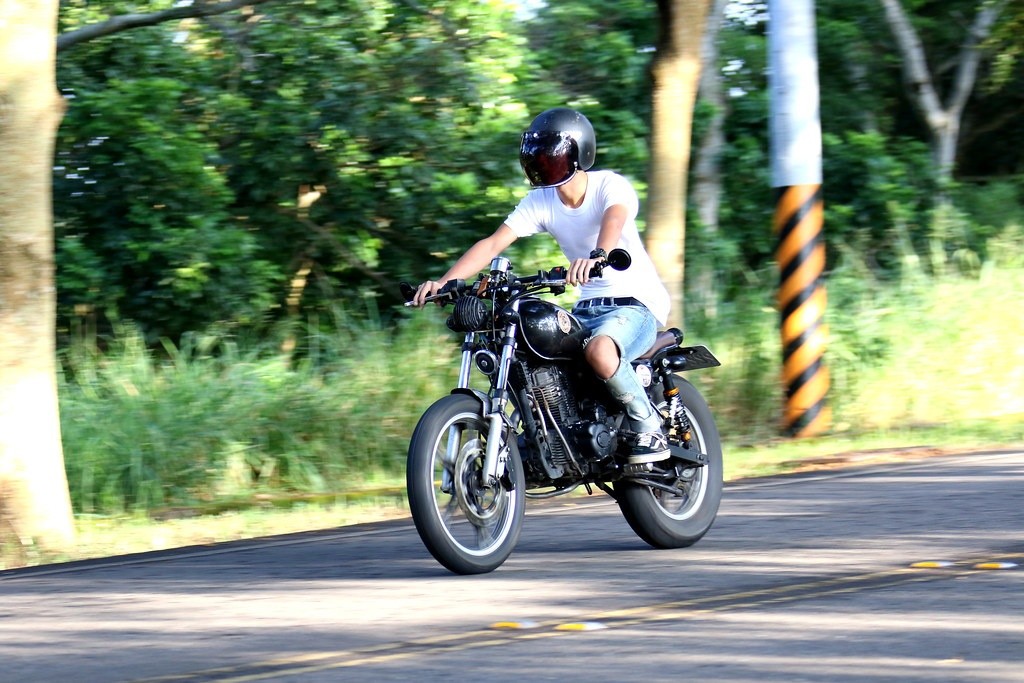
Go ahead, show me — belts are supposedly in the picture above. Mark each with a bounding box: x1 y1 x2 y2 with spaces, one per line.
577 297 645 307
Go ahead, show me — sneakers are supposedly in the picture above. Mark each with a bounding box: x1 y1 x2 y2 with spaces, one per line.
628 432 671 463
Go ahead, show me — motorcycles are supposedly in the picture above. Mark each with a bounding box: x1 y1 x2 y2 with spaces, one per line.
400 247 724 577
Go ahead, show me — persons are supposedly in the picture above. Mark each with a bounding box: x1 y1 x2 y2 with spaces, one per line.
413 108 673 464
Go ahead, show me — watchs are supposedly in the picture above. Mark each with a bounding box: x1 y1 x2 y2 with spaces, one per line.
589 247 609 262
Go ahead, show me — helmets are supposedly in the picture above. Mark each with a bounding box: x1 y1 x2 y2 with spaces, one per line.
520 108 596 189
